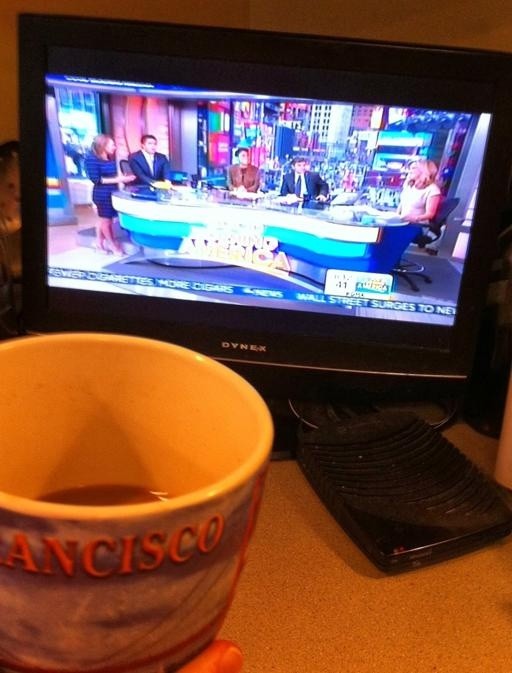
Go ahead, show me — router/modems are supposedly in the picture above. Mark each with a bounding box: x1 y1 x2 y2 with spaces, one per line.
294 409 512 575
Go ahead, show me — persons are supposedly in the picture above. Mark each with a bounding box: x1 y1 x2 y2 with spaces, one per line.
262 148 366 192
281 156 328 203
129 133 171 191
372 158 441 272
83 134 137 256
225 147 260 194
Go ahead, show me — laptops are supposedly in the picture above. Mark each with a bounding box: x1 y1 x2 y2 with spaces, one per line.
318 192 364 206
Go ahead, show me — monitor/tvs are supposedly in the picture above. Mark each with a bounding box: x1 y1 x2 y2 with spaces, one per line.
16 13 512 463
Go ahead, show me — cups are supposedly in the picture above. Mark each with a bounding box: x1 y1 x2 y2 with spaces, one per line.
0 330 277 673
